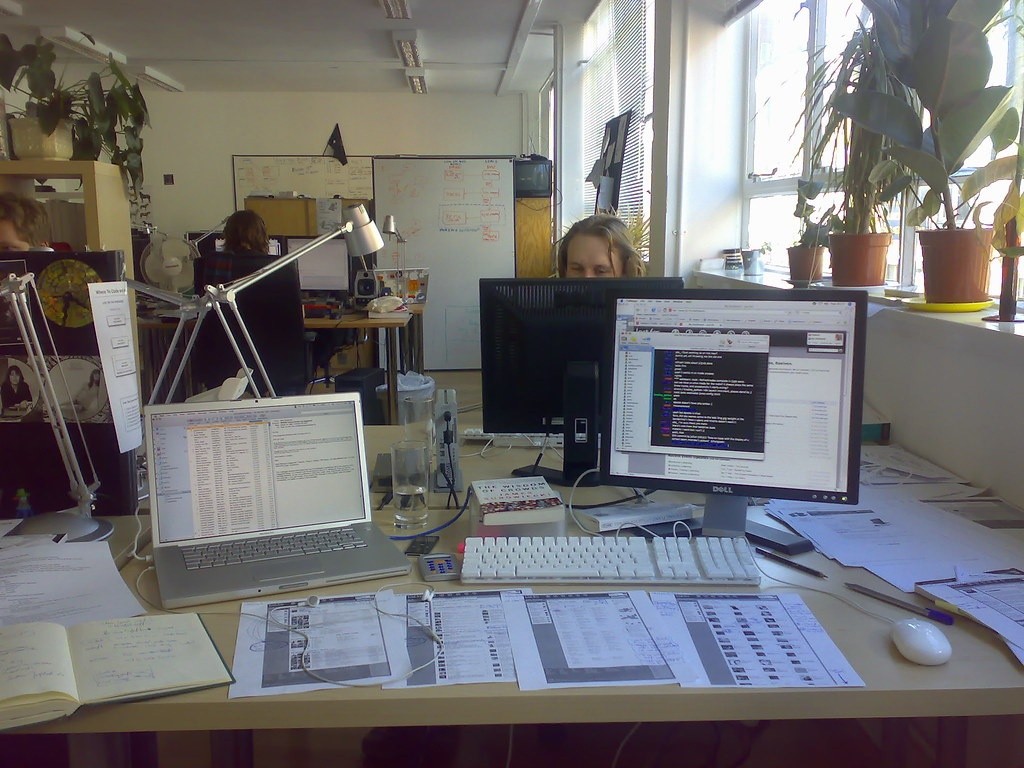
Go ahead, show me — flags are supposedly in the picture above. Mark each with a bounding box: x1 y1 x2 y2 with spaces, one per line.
329 124 348 166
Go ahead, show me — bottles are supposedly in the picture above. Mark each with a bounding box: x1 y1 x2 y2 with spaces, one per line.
14 489 34 517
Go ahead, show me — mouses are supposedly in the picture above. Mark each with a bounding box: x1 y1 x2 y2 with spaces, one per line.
330 312 342 320
891 617 952 665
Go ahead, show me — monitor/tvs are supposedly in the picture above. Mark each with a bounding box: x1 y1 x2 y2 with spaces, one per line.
283 235 352 304
479 275 868 556
514 160 552 198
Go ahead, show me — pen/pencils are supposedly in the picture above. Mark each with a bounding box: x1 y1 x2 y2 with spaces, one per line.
844 582 954 626
756 547 830 580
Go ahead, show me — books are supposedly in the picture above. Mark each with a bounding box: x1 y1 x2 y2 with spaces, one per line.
574 502 693 531
0 611 237 730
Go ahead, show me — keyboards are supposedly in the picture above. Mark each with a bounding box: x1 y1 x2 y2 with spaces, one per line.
465 427 564 448
462 536 761 587
304 308 330 319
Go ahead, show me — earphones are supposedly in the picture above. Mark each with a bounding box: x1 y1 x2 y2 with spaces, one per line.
420 588 436 601
298 595 320 607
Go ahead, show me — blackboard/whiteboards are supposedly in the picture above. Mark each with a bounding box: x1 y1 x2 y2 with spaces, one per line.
372 155 517 370
232 154 374 239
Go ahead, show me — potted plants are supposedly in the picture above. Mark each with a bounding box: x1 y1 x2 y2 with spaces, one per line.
788 1 1023 303
0 32 153 199
741 242 772 276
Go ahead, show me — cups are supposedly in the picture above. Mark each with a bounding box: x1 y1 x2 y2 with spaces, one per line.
388 441 431 530
401 396 433 443
723 249 743 270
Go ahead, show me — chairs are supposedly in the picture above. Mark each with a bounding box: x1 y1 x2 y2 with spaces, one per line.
310 327 368 388
193 255 317 394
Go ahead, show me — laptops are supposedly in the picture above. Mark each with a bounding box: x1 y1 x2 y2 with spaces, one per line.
144 391 412 610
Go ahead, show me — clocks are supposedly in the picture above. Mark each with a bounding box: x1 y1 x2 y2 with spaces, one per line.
36 258 102 328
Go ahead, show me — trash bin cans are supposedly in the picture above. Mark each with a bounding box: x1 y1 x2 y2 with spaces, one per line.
375 375 437 426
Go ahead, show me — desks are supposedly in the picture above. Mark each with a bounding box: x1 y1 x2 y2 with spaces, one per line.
0 425 1024 768
3 409 25 416
137 301 426 425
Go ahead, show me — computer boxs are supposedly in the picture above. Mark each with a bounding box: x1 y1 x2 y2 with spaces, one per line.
336 369 387 427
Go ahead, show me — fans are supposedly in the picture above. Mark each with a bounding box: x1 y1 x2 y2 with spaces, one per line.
140 237 203 295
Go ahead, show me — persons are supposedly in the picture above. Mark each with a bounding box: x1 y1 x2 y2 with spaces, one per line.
558 215 646 277
221 209 269 255
0 193 51 250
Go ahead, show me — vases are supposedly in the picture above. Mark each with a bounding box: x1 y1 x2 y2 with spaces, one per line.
725 248 741 268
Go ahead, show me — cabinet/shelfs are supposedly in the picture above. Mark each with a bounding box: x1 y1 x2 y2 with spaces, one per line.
515 197 552 277
244 198 370 237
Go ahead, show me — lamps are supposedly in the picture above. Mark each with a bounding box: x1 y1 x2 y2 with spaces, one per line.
143 221 169 241
0 273 114 545
381 214 407 306
206 203 384 399
184 215 231 259
406 67 429 94
138 67 186 92
379 0 412 19
392 29 423 67
0 0 22 16
40 25 126 65
125 278 213 500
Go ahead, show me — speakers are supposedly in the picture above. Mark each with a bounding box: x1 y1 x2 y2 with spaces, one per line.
355 270 379 306
